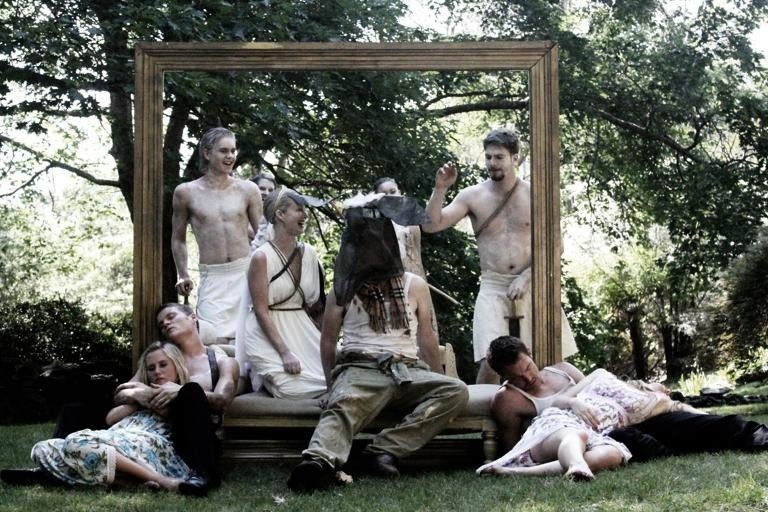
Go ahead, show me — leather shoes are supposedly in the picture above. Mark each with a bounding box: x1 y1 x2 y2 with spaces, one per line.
1 468 45 486
178 476 207 495
359 444 400 479
753 426 767 448
286 460 326 496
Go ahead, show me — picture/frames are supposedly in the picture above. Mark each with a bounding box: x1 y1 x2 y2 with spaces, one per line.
131 42 562 465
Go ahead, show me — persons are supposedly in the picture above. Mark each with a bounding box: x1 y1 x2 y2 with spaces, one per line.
421 127 580 385
247 174 279 250
1 302 241 495
373 176 440 346
475 368 712 479
288 222 469 494
486 335 768 460
170 127 271 351
235 188 346 400
30 341 192 491
